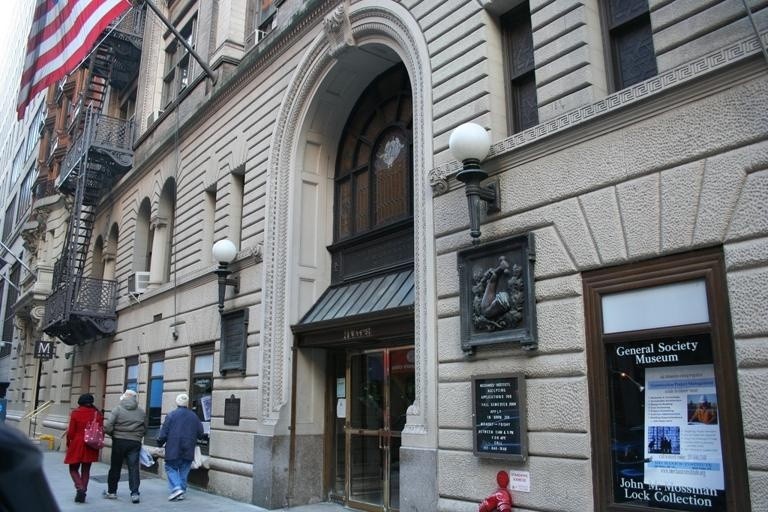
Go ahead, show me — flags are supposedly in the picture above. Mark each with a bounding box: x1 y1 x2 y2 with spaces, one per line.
16 1 137 125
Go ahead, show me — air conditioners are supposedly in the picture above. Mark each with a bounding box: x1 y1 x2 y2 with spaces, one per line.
145 109 164 130
125 269 152 297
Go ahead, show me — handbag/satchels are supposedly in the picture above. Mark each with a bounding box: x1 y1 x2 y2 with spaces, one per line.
139 446 155 467
190 445 202 469
84 411 104 450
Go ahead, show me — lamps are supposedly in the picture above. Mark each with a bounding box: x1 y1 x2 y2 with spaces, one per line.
448 121 503 246
210 239 241 316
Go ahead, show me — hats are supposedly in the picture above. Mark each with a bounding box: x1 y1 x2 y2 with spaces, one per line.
176 393 189 407
77 394 95 405
120 390 137 401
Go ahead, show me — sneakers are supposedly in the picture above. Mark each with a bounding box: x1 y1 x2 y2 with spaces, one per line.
74 490 86 503
104 490 117 499
167 488 186 501
131 494 140 504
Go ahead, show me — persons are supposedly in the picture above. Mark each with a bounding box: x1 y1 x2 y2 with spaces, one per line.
64 392 104 502
0 413 60 512
104 389 148 504
158 393 205 501
